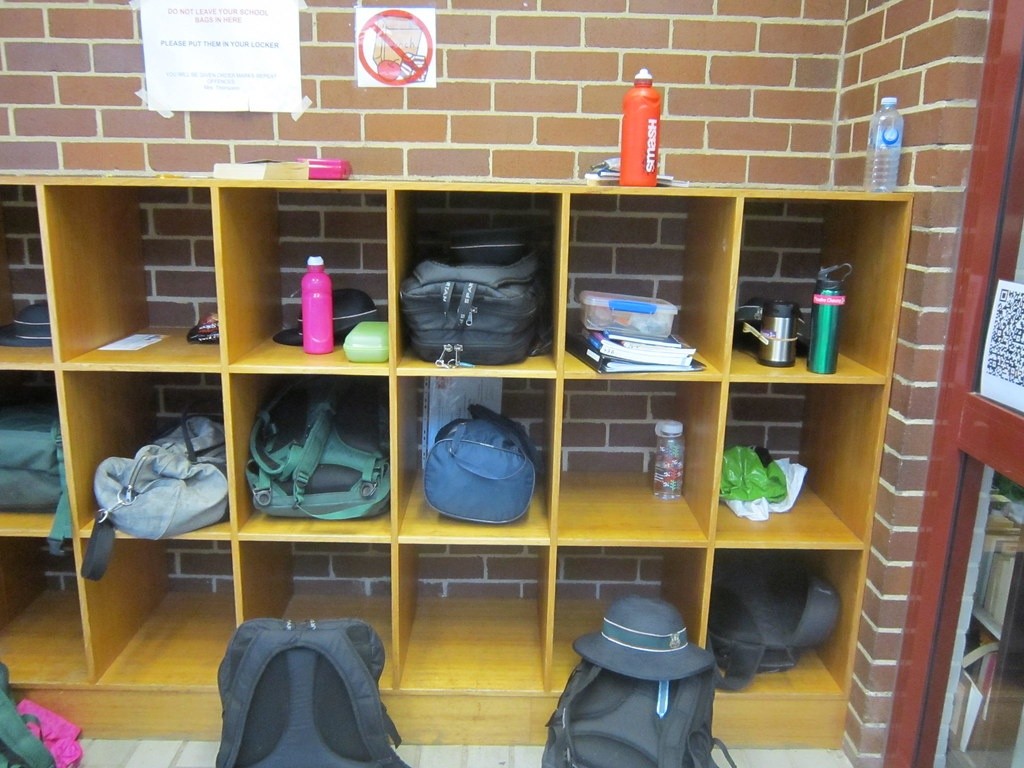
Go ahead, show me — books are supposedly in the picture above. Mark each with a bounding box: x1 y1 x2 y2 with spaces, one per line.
213 158 310 181
584 170 690 188
581 328 697 367
946 492 1022 720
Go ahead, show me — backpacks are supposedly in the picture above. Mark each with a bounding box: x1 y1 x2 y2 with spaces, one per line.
215 616 414 768
704 558 842 695
1 408 74 556
395 255 554 371
0 663 59 768
243 378 392 525
541 655 737 768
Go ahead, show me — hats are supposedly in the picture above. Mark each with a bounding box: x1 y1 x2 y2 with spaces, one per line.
573 594 717 685
273 286 379 349
1 300 53 352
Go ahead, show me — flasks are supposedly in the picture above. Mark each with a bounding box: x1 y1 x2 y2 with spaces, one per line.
757 299 799 367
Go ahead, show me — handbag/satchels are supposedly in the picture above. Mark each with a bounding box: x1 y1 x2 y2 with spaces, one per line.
422 400 540 525
79 397 230 583
13 698 84 768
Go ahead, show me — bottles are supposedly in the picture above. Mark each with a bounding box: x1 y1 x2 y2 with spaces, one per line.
617 68 661 188
807 263 852 373
863 97 903 193
652 420 684 500
302 257 333 353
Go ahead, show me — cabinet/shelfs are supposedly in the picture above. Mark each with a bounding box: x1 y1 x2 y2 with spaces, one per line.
1 174 915 749
945 483 1023 768
